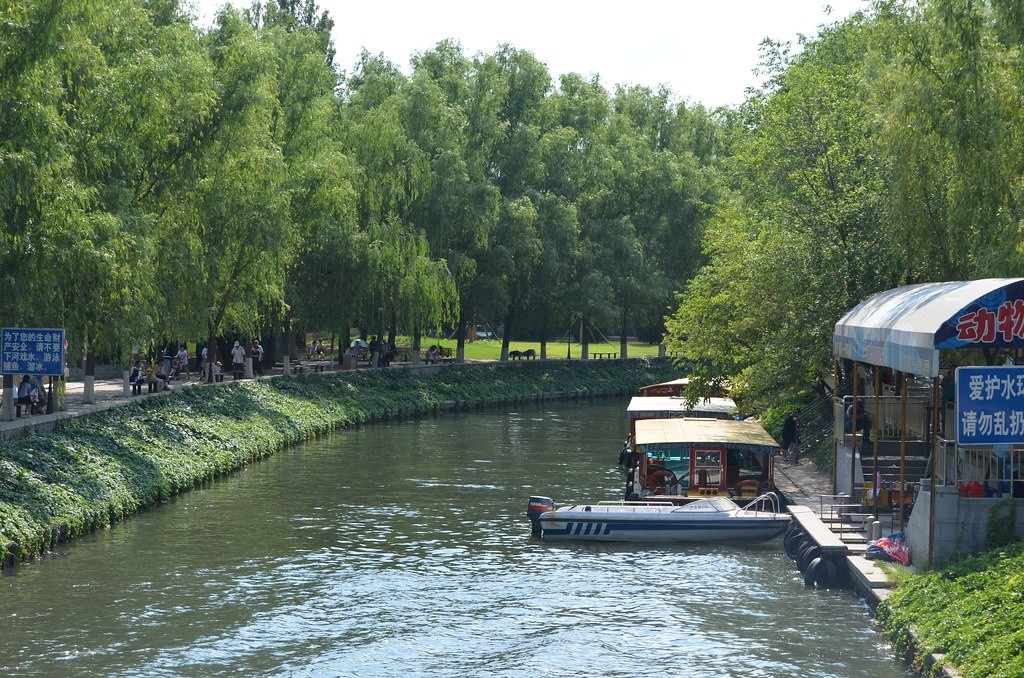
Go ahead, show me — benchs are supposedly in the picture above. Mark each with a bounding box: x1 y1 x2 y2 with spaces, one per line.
14 401 42 419
306 363 330 373
231 370 245 379
508 354 539 360
130 379 157 395
667 350 686 356
215 373 227 383
590 352 618 359
392 348 410 362
351 347 368 360
306 347 326 361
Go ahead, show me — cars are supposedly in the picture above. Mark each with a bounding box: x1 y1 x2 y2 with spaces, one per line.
476 325 493 340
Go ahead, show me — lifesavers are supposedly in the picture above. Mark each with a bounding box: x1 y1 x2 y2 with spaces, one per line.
782 520 828 588
649 470 677 495
735 479 764 496
625 468 635 500
618 432 631 466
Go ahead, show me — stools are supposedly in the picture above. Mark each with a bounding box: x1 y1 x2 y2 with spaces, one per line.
294 365 302 374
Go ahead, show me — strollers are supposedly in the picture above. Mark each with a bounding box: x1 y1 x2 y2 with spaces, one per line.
168 357 185 381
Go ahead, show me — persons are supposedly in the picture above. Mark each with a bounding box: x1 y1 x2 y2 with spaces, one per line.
13 374 48 415
252 340 264 377
844 399 870 446
380 339 391 367
231 341 246 379
202 344 208 358
175 344 190 380
215 363 221 373
428 345 446 360
941 364 957 431
863 364 873 386
367 336 376 366
129 360 170 395
346 342 360 354
883 368 892 385
310 339 322 355
782 407 802 461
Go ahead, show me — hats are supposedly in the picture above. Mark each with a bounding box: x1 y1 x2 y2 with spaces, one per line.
858 399 864 406
217 361 222 366
234 341 239 347
254 340 259 344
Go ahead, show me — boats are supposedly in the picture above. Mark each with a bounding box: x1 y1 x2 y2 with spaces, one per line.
626 417 781 511
618 396 740 463
527 492 791 542
638 376 748 404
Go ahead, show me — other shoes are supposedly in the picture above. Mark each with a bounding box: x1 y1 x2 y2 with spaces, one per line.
863 438 873 446
783 456 788 461
